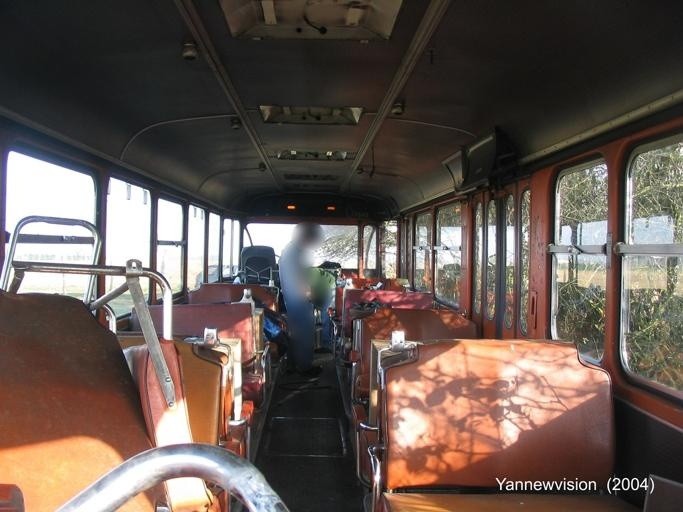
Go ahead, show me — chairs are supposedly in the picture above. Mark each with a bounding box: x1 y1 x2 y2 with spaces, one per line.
239 247 279 286
